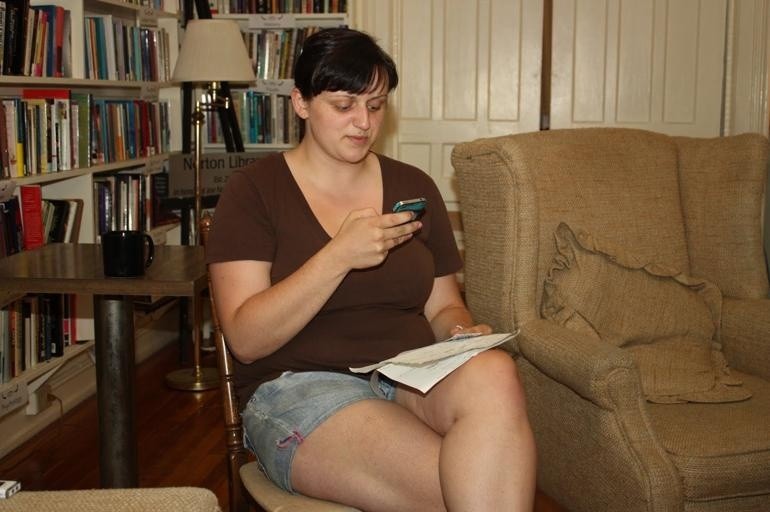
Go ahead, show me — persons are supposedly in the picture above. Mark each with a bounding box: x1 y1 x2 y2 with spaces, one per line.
209 29 539 512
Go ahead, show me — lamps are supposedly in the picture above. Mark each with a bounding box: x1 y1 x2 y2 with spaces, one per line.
164 15 258 388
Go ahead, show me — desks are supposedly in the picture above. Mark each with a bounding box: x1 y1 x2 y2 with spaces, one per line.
3 242 212 488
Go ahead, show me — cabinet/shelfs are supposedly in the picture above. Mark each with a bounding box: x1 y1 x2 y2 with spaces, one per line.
0 1 179 453
181 0 349 252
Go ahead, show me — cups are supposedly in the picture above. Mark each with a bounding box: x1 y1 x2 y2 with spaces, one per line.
99 229 155 278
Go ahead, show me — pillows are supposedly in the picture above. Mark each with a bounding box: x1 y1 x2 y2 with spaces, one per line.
538 224 754 405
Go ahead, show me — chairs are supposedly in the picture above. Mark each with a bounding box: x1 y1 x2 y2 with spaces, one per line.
447 126 768 506
200 261 367 512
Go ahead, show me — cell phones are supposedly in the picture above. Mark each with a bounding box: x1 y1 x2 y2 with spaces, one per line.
393 198 427 221
